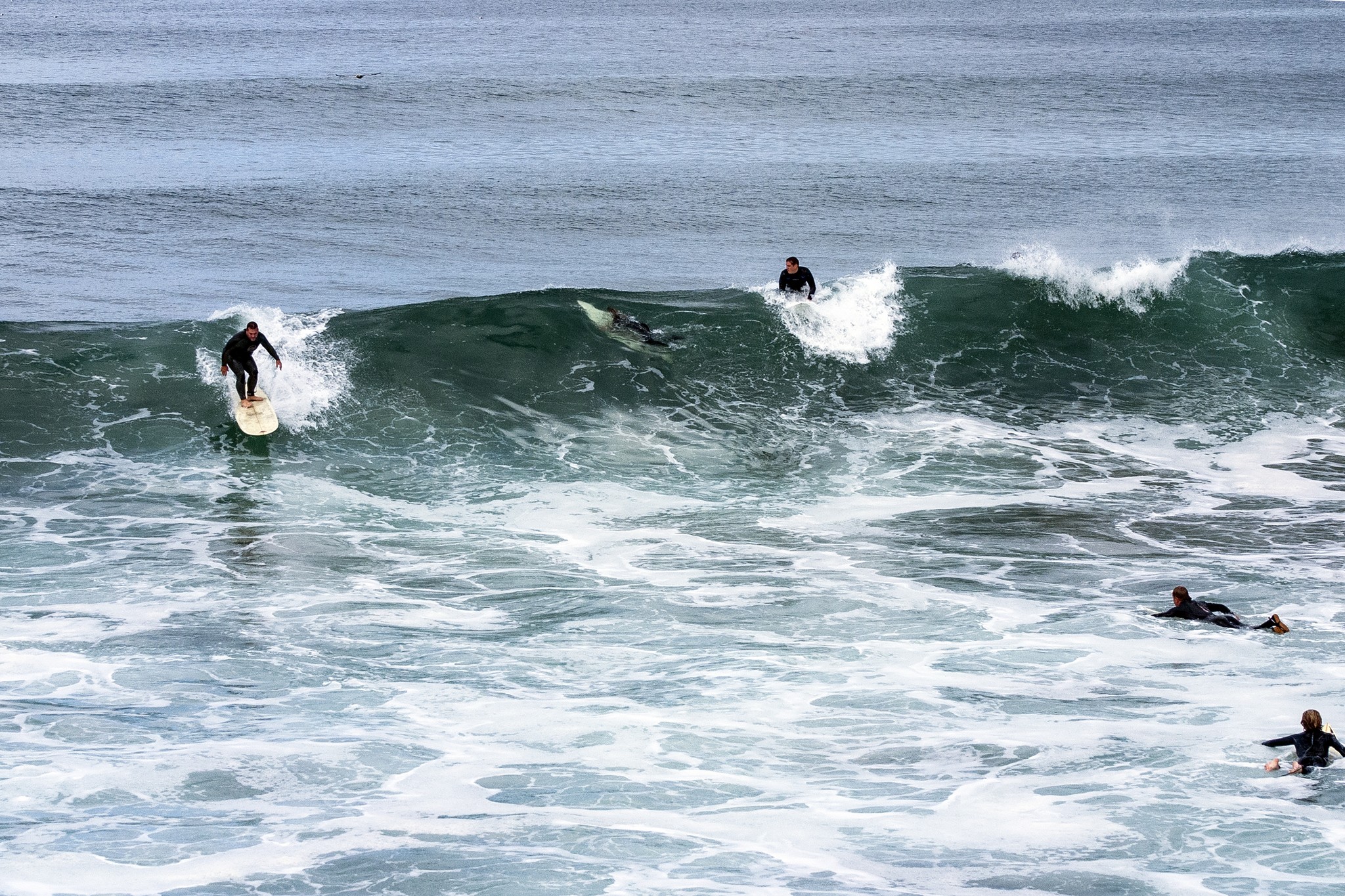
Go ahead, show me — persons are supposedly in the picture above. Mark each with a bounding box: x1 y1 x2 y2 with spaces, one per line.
779 257 816 300
220 322 282 408
1151 586 1289 634
605 306 686 347
1262 710 1345 775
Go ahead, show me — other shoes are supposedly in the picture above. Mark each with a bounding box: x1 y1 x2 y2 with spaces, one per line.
1272 625 1285 634
1271 614 1290 632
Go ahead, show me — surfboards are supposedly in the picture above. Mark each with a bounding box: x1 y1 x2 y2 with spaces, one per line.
795 303 808 306
1320 723 1339 755
226 374 279 436
576 299 672 363
